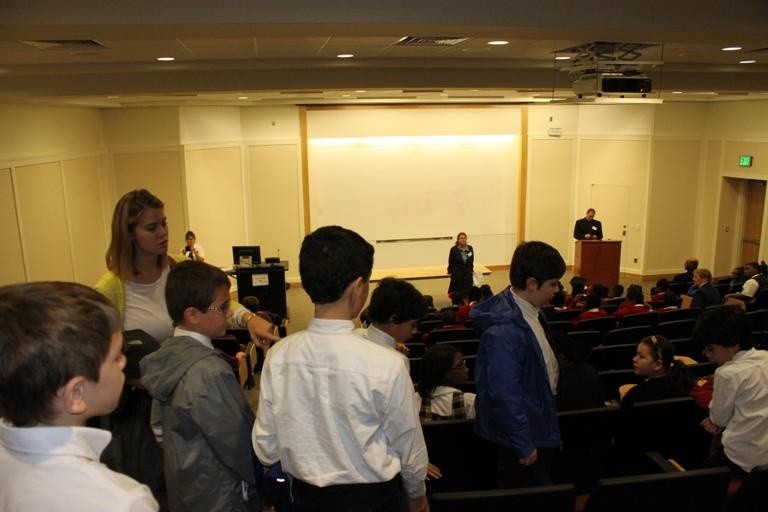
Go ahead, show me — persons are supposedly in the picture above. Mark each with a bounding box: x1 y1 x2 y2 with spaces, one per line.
92 189 282 349
361 258 768 512
469 240 566 487
574 208 603 240
447 233 474 306
0 280 161 512
179 230 205 263
250 226 430 512
138 260 263 512
241 296 273 324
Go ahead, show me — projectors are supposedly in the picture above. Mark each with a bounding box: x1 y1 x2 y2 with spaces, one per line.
572 72 653 98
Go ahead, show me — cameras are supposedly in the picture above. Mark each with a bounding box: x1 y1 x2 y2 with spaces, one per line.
185 245 192 251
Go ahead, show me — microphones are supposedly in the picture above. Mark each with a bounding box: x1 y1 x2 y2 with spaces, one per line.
278 248 280 259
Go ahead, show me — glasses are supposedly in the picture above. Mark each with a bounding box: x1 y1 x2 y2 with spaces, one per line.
448 359 466 370
203 301 231 316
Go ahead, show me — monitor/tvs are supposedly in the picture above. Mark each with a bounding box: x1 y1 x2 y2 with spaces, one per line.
232 245 261 269
